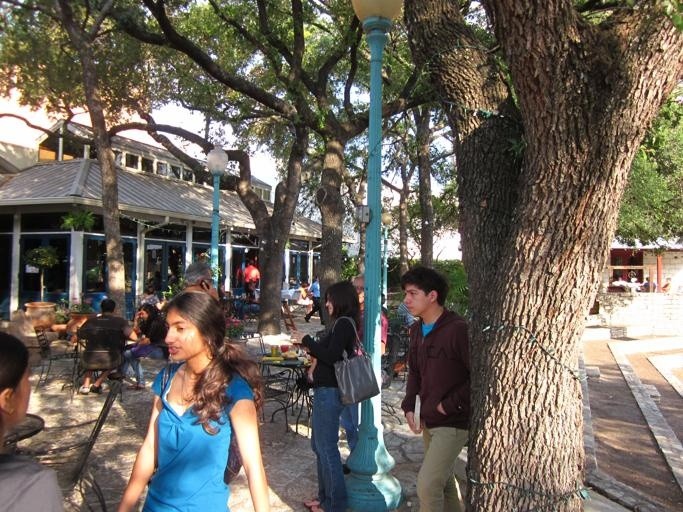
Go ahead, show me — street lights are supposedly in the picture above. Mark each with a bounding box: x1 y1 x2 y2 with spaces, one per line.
205 140 230 305
379 212 394 313
338 0 405 509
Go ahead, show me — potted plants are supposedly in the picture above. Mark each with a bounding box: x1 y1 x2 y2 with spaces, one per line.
59 208 100 234
19 247 62 332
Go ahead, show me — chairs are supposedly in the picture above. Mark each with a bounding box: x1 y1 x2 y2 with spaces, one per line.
33 325 138 405
236 331 291 425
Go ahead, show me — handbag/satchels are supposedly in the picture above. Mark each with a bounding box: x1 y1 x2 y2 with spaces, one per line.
330 316 379 405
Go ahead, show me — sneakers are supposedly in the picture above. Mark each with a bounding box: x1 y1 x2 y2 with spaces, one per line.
79 386 89 393
127 384 145 390
305 500 324 512
89 384 103 393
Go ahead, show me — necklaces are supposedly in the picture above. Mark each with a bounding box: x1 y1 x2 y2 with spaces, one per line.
182 364 192 406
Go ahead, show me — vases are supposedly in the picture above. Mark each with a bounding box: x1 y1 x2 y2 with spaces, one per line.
64 312 88 343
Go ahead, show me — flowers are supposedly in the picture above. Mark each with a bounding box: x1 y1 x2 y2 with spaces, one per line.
70 292 93 315
224 316 244 337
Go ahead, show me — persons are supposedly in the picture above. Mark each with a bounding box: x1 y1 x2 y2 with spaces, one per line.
608 275 672 292
138 257 417 391
115 292 270 512
69 299 137 396
109 303 166 390
179 261 219 304
289 278 360 511
399 265 471 511
340 274 389 477
0 330 65 511
